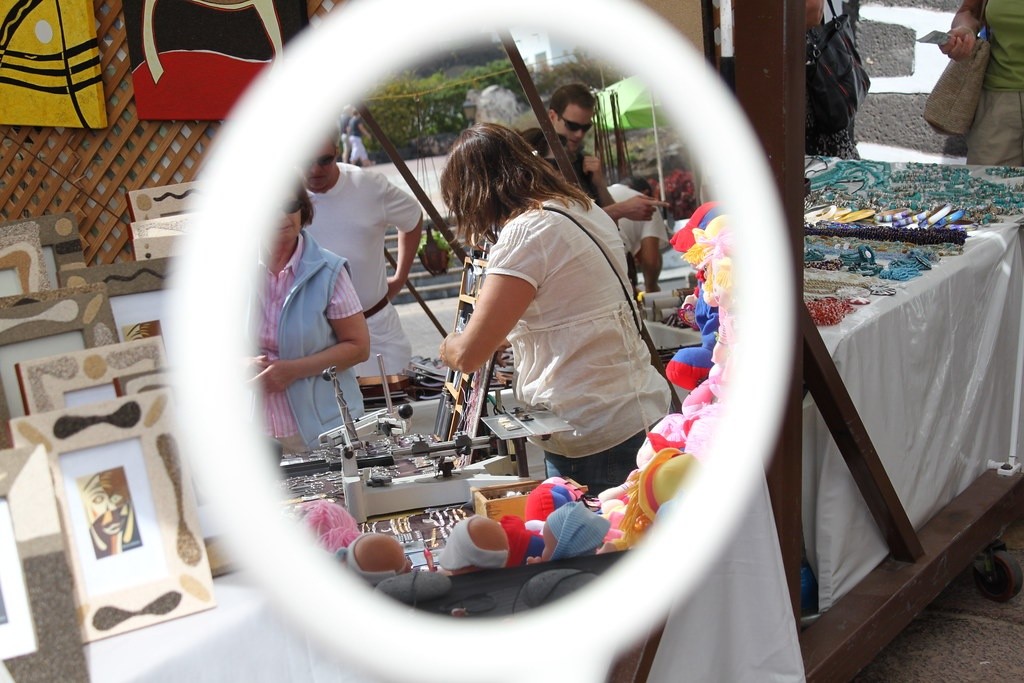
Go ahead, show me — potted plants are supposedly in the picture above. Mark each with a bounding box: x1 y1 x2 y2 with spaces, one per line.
417 225 452 276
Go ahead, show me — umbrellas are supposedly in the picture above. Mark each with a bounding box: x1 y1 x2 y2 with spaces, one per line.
589 78 681 202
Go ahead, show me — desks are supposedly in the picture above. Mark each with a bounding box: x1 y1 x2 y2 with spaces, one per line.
642 156 1024 683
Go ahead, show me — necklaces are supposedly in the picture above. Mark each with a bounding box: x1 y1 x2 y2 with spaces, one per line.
798 153 1023 328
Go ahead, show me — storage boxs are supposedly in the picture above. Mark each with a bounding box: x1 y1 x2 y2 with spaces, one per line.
472 476 589 523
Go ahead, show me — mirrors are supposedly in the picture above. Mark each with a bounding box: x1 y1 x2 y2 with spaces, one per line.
162 0 800 683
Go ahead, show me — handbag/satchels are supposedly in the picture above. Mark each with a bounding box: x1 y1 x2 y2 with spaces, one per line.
923 0 991 134
806 0 870 136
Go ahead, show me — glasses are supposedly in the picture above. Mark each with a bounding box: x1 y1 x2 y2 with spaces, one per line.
284 200 302 213
308 147 336 166
561 116 592 132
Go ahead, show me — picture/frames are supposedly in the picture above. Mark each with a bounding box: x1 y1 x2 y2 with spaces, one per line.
0 182 245 683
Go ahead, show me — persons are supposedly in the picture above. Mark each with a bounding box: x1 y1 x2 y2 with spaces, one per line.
298 203 736 622
717 0 861 160
937 0 1024 166
250 77 707 493
